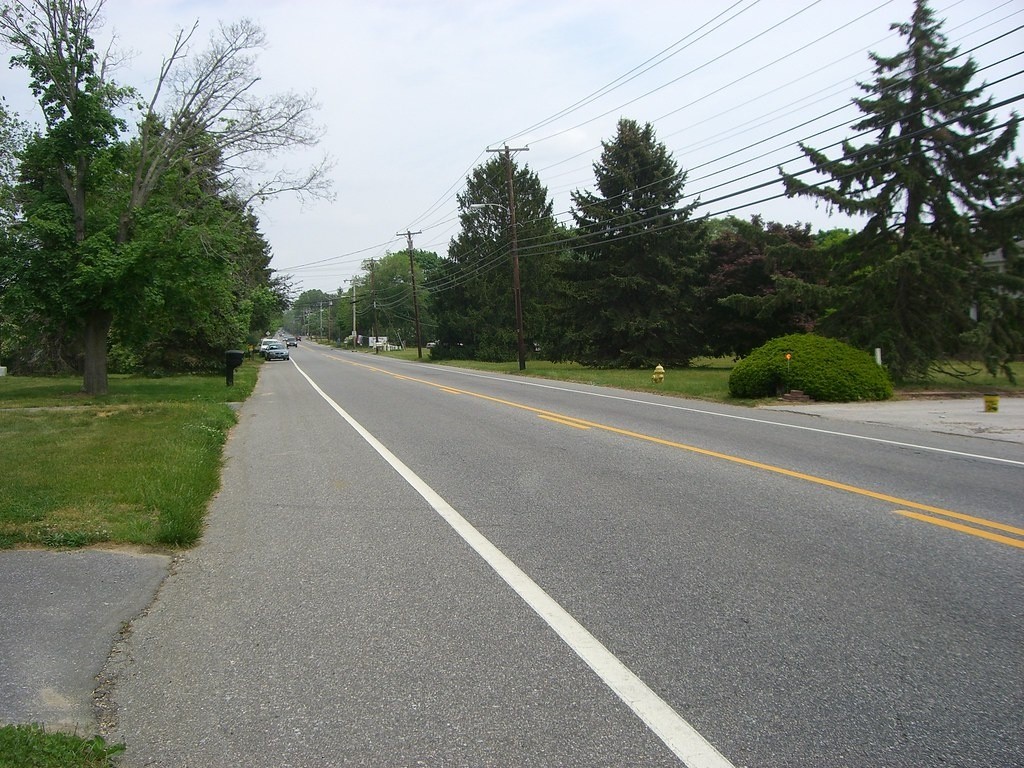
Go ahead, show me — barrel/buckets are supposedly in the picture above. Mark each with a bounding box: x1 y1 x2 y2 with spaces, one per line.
985 394 999 412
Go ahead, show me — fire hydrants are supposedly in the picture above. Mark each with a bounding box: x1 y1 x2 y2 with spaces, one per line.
651 364 665 384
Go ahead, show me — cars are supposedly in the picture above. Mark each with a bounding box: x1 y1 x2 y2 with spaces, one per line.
264 343 289 361
259 338 280 357
279 329 302 348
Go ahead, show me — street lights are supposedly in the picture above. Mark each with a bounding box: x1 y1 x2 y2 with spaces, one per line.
470 201 526 371
